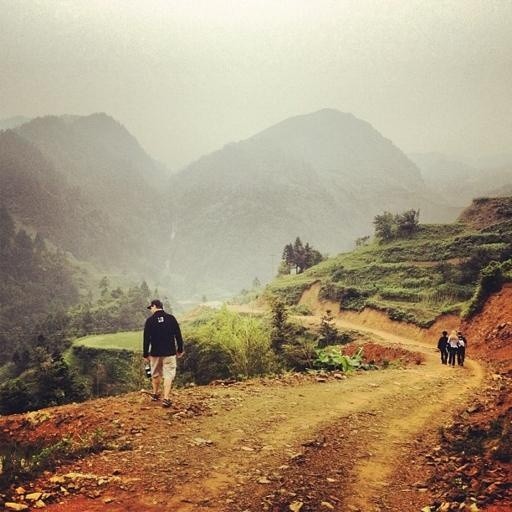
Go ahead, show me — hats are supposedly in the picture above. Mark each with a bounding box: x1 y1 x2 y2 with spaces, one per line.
147 300 163 310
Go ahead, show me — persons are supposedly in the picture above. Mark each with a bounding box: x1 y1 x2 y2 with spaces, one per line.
142 298 186 407
437 331 449 365
446 330 459 368
457 331 467 366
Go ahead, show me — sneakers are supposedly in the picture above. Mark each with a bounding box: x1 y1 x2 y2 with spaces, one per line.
162 398 171 407
151 393 159 401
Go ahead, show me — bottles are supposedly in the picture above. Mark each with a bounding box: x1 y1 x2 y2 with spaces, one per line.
145 363 151 378
